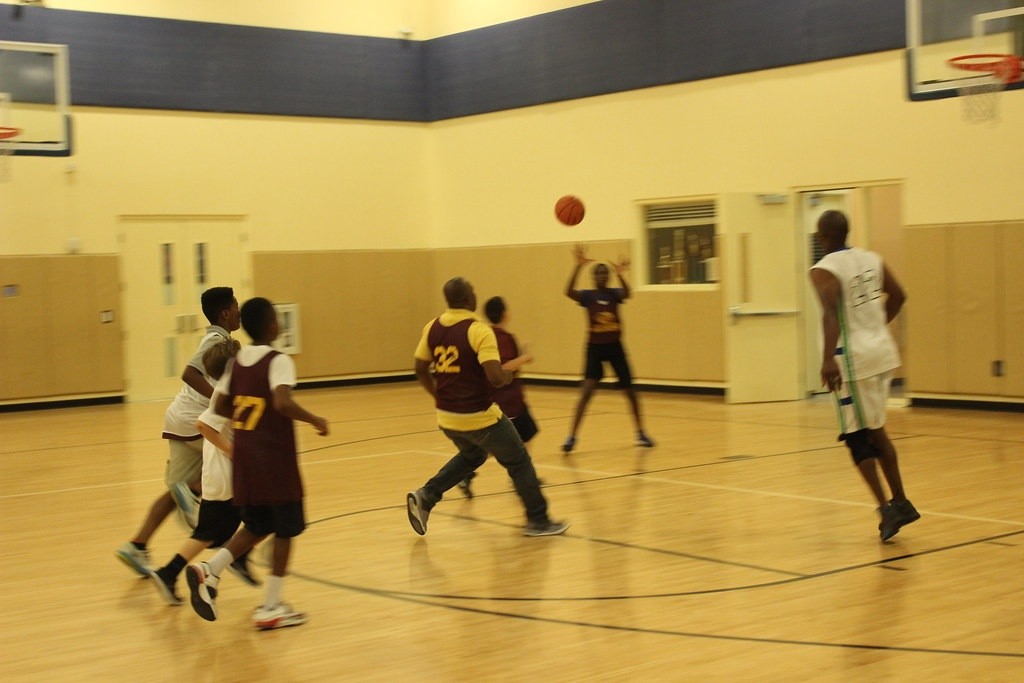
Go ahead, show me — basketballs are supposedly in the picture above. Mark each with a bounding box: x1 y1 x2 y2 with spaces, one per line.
555 196 585 226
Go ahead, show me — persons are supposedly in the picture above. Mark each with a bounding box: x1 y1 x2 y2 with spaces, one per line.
149 339 264 606
457 297 540 500
114 286 241 577
406 276 572 537
560 242 656 454
184 297 329 631
809 210 922 542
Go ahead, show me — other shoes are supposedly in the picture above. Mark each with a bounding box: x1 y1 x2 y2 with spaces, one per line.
635 435 652 446
877 506 904 542
562 438 574 450
116 543 152 575
172 481 201 530
887 488 920 526
151 567 184 605
229 554 257 585
458 472 477 498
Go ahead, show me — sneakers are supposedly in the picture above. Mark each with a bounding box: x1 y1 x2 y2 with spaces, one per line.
253 602 306 628
187 561 220 621
408 487 432 535
522 520 570 536
